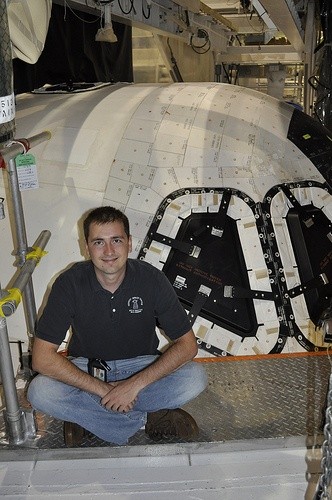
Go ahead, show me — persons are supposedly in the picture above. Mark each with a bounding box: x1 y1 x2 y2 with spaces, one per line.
27 207 210 446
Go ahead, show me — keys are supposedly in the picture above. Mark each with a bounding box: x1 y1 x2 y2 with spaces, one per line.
96 359 111 372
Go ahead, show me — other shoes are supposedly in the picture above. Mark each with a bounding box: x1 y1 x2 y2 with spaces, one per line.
63 421 94 447
145 408 198 443
